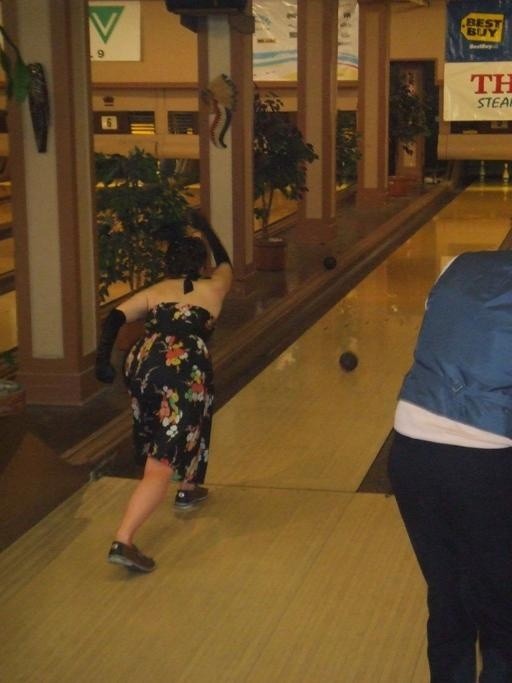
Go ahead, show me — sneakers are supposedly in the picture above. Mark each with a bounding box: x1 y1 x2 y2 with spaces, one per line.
108 540 155 573
174 484 208 510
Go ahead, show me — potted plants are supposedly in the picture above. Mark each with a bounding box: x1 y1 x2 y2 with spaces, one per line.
93 145 195 352
252 87 318 272
336 107 363 207
1 352 24 418
387 61 435 196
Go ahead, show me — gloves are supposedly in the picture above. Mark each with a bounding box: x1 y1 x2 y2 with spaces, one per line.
190 212 232 268
96 308 124 383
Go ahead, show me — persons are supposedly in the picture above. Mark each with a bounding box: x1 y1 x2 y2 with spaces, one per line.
94 208 237 575
386 249 512 682
